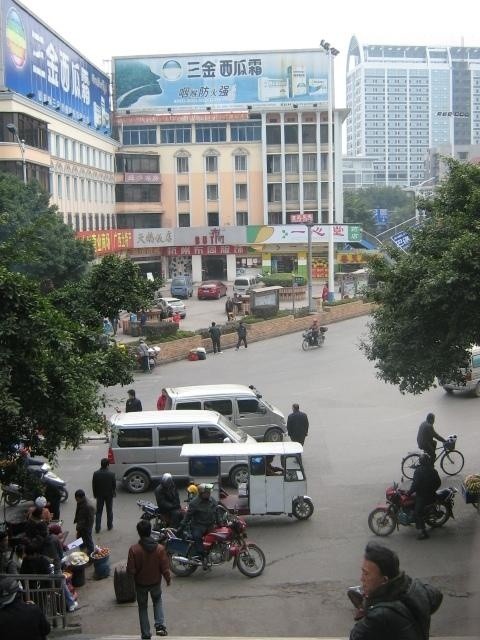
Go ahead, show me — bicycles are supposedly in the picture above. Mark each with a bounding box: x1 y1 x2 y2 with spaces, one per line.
400 433 466 478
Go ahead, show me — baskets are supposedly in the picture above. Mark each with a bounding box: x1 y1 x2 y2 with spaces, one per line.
447 440 455 450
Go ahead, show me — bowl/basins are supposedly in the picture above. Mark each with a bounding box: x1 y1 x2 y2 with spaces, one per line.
90 548 112 563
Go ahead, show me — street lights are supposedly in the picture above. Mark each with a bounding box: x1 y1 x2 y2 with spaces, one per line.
304 219 314 311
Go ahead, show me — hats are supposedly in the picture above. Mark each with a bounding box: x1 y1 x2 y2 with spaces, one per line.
49 525 62 534
1 577 26 605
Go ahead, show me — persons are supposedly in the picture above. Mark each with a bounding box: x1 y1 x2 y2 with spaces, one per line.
126 389 142 413
307 319 320 345
157 389 167 411
407 452 441 540
322 285 328 302
255 455 288 476
287 403 309 464
349 544 443 640
417 413 447 462
102 293 248 371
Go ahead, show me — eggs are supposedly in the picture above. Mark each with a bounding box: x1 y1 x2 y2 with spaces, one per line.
94 549 110 558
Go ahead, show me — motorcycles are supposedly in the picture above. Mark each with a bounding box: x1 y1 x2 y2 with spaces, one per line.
136 496 194 544
1 429 69 507
170 502 268 578
179 442 315 521
125 344 161 370
300 326 328 351
366 481 456 537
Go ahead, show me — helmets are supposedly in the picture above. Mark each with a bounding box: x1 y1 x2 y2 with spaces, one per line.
162 472 172 484
187 484 198 495
419 454 429 464
35 496 47 508
198 483 214 494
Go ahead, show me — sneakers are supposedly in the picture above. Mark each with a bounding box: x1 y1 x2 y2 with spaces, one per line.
156 625 167 636
69 601 78 612
418 532 427 539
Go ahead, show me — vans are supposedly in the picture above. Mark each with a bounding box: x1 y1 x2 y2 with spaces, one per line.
232 275 258 297
438 345 480 397
157 384 286 444
108 409 262 495
171 276 195 299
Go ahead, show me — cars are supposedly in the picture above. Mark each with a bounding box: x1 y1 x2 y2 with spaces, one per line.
197 280 229 301
150 297 187 320
235 263 246 272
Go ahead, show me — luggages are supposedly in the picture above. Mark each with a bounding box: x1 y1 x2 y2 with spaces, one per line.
114 566 136 603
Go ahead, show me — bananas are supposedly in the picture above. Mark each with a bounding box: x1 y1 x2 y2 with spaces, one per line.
464 474 480 495
